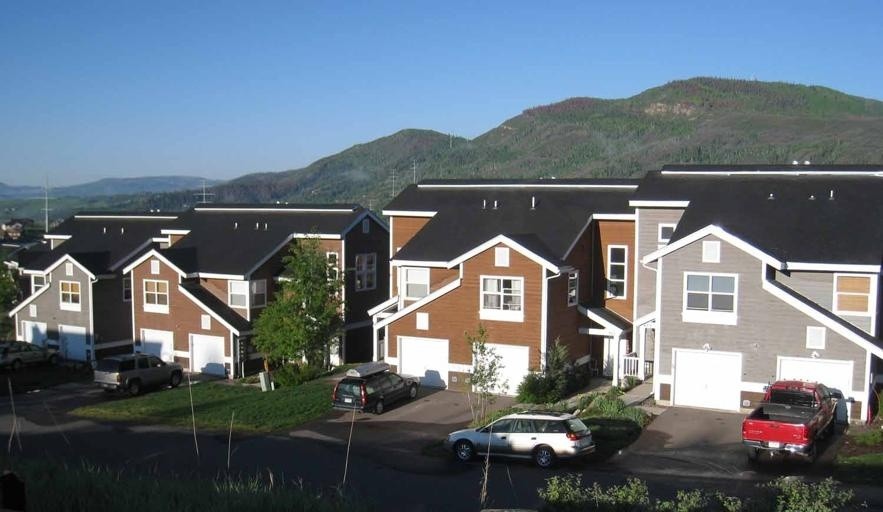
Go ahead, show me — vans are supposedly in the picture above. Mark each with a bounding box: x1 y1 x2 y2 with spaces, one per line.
332 362 421 416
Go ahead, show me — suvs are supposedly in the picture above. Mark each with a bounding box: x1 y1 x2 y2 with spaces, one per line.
448 407 596 469
93 351 184 396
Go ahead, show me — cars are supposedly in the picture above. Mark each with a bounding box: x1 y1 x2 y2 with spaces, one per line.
0 341 60 373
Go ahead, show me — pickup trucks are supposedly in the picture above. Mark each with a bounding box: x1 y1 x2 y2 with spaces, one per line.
741 378 842 467
